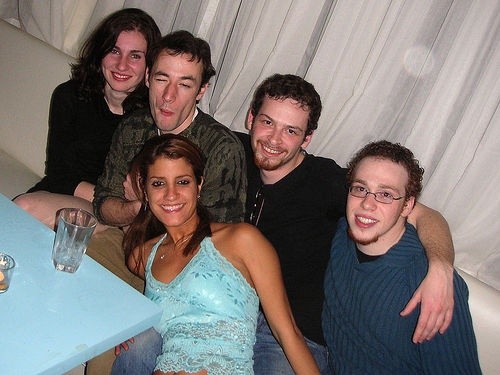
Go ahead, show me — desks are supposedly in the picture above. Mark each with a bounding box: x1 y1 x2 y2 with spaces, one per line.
0 190 164 375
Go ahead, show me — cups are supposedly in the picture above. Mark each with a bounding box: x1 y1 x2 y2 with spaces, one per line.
52 207 97 273
0 253 16 294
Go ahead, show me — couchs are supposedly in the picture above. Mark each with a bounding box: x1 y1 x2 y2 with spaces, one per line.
0 18 500 375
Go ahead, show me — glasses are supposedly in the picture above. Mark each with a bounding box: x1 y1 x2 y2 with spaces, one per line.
349 185 406 204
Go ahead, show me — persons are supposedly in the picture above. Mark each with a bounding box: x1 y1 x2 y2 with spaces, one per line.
114 133 322 375
319 140 482 375
109 73 455 375
11 7 164 236
84 29 247 375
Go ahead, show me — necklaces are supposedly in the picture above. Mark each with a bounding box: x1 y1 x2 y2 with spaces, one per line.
161 234 193 260
